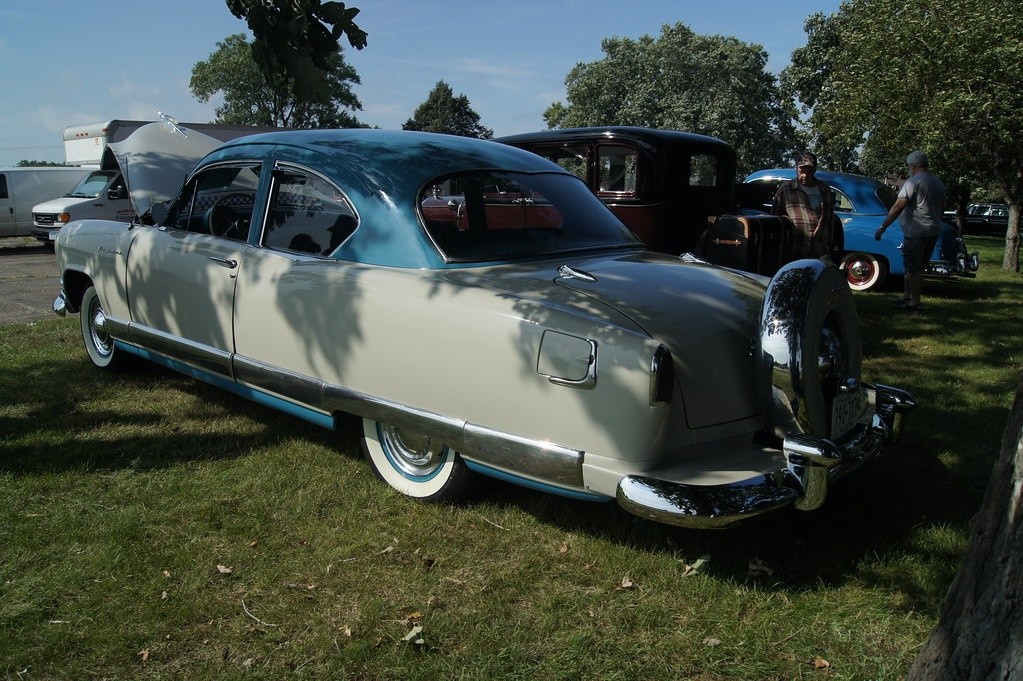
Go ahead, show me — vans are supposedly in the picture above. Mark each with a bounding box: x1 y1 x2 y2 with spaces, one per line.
0 167 91 239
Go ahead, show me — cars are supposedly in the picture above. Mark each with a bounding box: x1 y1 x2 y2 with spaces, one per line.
54 120 923 533
421 125 844 278
741 168 980 293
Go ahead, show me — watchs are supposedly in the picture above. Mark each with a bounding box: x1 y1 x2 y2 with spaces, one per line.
880 226 886 230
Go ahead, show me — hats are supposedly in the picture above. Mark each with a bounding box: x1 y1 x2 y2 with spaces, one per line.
795 151 817 170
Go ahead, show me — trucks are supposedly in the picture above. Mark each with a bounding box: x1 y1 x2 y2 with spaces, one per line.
31 118 301 254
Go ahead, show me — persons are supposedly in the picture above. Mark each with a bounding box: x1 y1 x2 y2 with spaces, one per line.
770 152 834 263
875 150 948 311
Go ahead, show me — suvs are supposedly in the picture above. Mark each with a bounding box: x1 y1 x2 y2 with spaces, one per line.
944 202 1010 225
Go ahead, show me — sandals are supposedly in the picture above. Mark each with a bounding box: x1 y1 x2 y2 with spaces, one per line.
894 297 911 305
898 303 923 312
